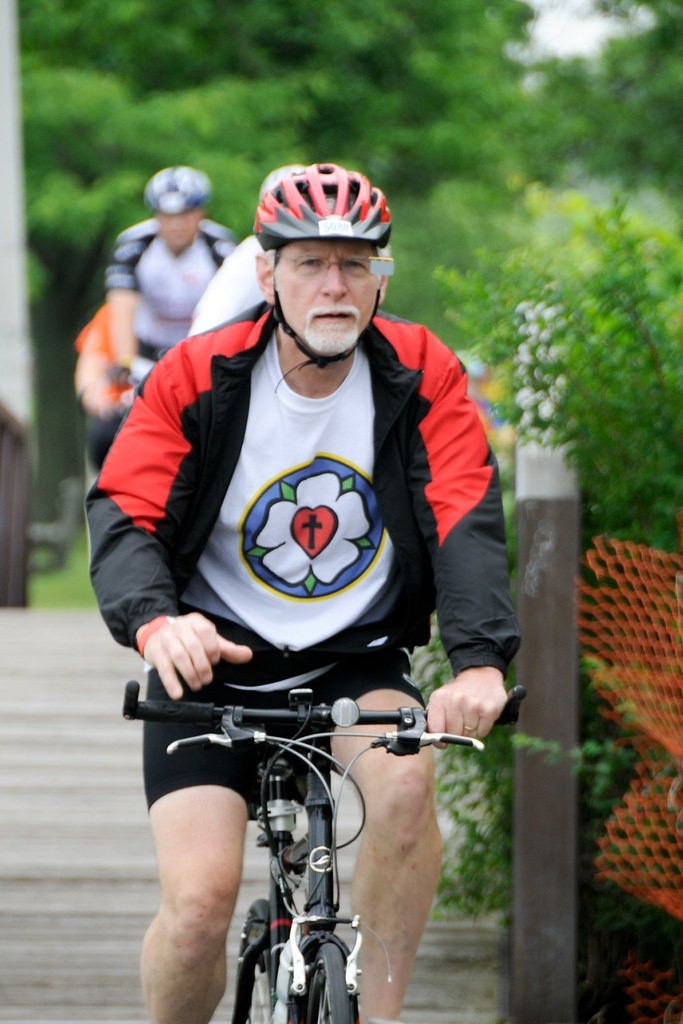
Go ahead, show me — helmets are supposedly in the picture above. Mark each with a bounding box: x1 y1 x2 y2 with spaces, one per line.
253 164 391 252
145 165 210 216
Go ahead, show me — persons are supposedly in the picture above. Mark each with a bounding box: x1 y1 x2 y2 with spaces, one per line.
84 165 236 470
188 234 263 340
82 162 522 1022
72 299 134 419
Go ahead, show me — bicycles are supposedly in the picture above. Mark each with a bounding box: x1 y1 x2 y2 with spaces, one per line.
120 678 535 1024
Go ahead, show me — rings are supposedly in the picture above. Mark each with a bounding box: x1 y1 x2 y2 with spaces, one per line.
463 725 477 731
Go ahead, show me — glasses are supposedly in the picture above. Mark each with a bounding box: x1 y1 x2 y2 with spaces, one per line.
276 254 380 279
